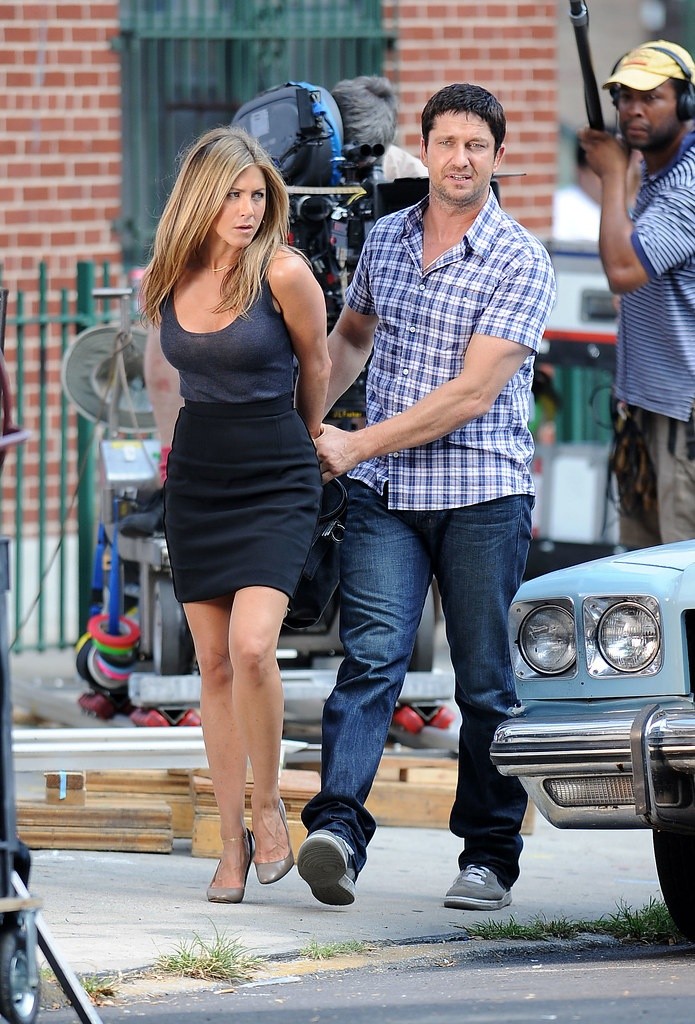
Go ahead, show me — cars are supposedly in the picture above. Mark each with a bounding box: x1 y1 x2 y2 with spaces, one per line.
488 536 695 943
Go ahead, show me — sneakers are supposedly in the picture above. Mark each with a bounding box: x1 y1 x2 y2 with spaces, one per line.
443 864 512 911
296 829 356 905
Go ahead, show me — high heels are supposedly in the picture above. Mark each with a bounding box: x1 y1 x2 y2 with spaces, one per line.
206 828 255 904
253 799 294 885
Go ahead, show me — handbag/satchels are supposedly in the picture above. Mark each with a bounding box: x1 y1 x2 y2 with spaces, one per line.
283 468 352 630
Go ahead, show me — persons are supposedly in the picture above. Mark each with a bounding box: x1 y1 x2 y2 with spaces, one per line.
139 130 333 904
579 42 694 551
556 130 640 238
335 75 431 184
298 83 555 910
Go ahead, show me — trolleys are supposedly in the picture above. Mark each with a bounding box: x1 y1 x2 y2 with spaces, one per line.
0 350 107 1024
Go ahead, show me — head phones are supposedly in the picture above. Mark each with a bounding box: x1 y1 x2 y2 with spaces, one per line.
608 47 695 122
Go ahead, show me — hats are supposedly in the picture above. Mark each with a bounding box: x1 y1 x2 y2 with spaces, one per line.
602 40 695 91
